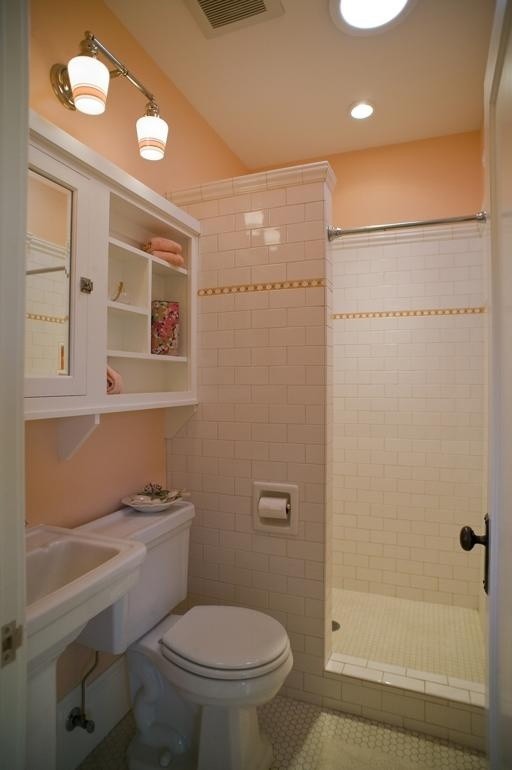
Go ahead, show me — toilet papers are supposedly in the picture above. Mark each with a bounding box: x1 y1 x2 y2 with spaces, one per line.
257 496 289 520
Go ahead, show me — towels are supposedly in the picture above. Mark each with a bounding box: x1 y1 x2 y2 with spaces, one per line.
107 366 124 394
142 237 182 253
153 251 184 266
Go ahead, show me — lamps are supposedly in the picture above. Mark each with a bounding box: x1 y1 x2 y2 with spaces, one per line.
50 31 170 160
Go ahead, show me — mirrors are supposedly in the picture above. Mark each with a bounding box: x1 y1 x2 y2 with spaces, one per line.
26 165 75 377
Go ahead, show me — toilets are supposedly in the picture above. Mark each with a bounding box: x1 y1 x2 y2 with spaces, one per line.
69 496 293 770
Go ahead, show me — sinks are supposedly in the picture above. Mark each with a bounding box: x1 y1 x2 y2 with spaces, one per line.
26 523 147 678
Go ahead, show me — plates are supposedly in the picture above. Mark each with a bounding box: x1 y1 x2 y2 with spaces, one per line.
118 491 182 514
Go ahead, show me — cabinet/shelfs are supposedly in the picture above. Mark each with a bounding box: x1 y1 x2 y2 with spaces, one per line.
25 112 202 421
